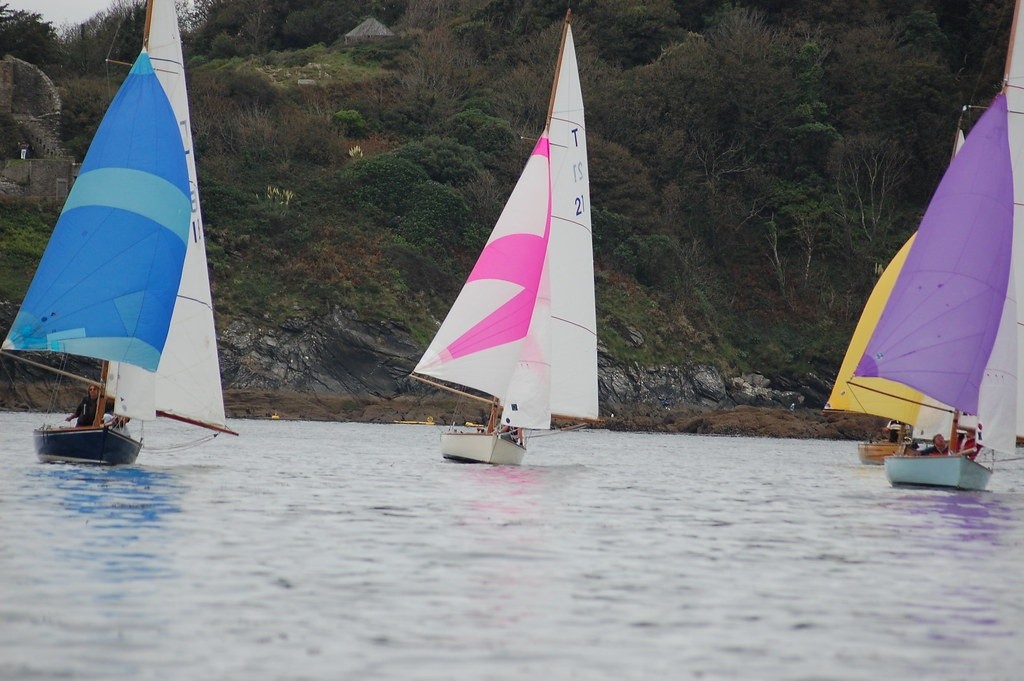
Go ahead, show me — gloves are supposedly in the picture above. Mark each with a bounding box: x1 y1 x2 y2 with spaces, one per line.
65 415 74 422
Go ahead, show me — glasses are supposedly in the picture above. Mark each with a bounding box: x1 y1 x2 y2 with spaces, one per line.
89 387 97 392
966 435 972 439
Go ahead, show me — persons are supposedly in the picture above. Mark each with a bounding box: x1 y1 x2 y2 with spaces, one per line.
103 413 131 438
883 419 901 443
491 426 523 447
65 384 114 428
913 434 948 456
956 429 976 460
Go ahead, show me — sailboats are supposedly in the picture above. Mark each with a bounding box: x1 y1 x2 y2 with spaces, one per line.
409 8 604 467
821 1 1024 491
0 0 239 467
